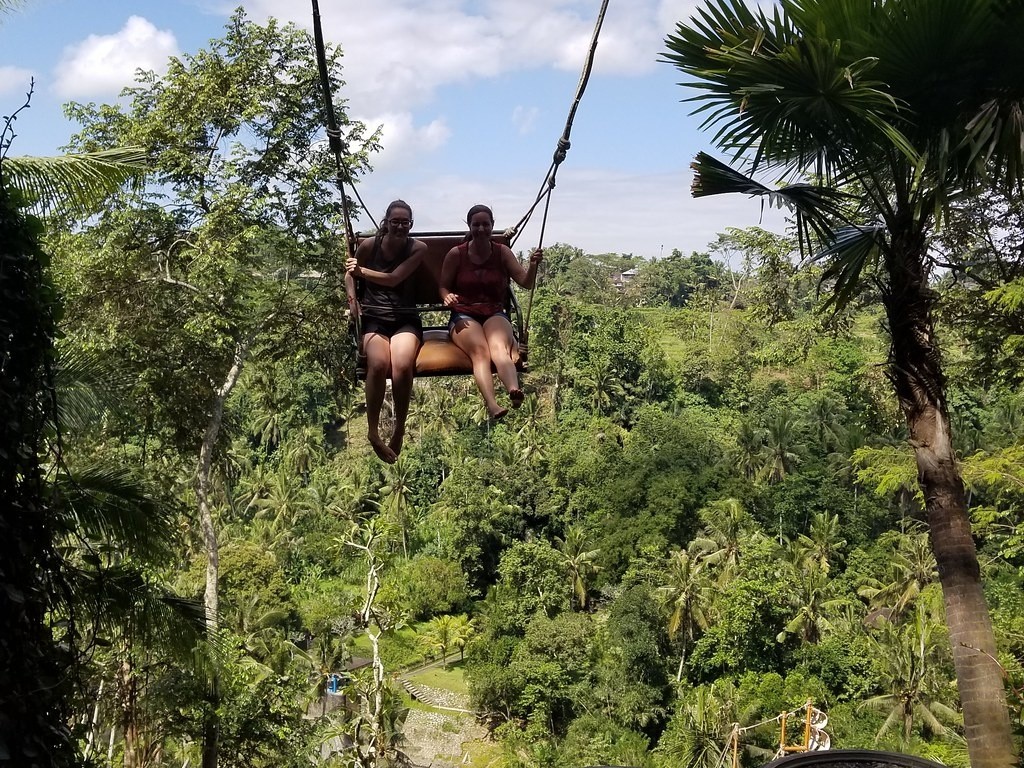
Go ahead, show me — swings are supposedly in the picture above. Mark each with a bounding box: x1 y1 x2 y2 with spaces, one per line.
307 0 616 375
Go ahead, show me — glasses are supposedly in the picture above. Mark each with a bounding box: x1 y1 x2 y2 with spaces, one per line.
385 219 412 228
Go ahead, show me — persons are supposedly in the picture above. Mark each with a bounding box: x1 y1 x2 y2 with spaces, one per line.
438 205 543 418
345 200 428 465
